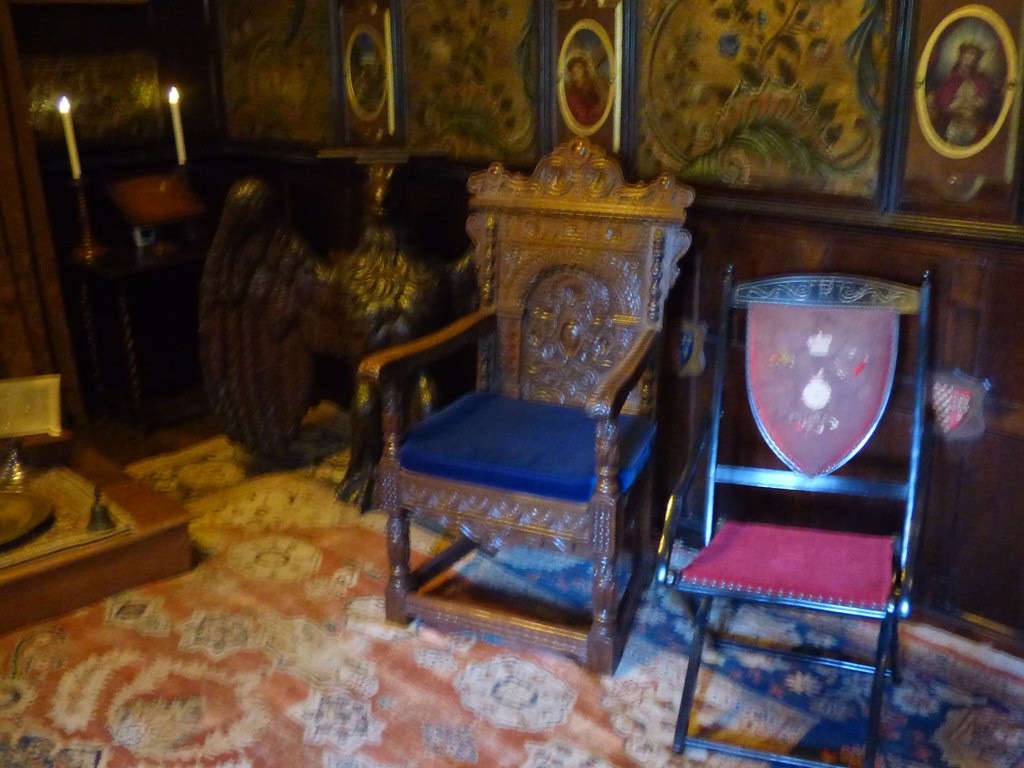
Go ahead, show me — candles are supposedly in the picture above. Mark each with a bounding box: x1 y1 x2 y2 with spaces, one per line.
170 100 186 165
62 105 81 180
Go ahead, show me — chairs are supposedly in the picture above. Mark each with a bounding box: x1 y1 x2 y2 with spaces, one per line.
356 137 695 678
676 264 936 768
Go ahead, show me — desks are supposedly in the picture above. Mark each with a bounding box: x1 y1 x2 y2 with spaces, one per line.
191 147 447 509
